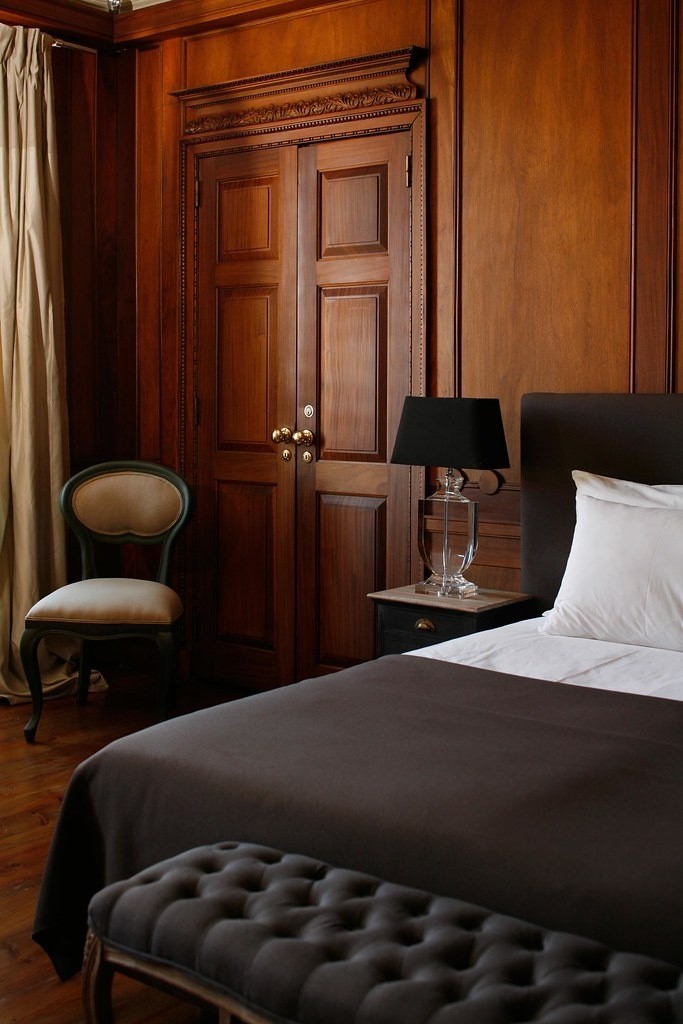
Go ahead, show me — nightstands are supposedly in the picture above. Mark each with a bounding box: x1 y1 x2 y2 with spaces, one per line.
367 576 541 657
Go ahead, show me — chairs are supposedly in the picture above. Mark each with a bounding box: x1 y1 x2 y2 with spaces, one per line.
21 462 195 739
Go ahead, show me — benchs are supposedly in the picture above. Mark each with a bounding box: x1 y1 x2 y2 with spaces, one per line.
78 839 681 1024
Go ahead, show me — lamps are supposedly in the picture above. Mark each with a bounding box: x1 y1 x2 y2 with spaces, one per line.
386 394 513 600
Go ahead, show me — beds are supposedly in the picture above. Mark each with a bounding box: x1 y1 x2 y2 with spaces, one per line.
34 388 683 987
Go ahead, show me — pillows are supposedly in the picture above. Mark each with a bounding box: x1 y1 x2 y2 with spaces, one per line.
543 471 683 652
